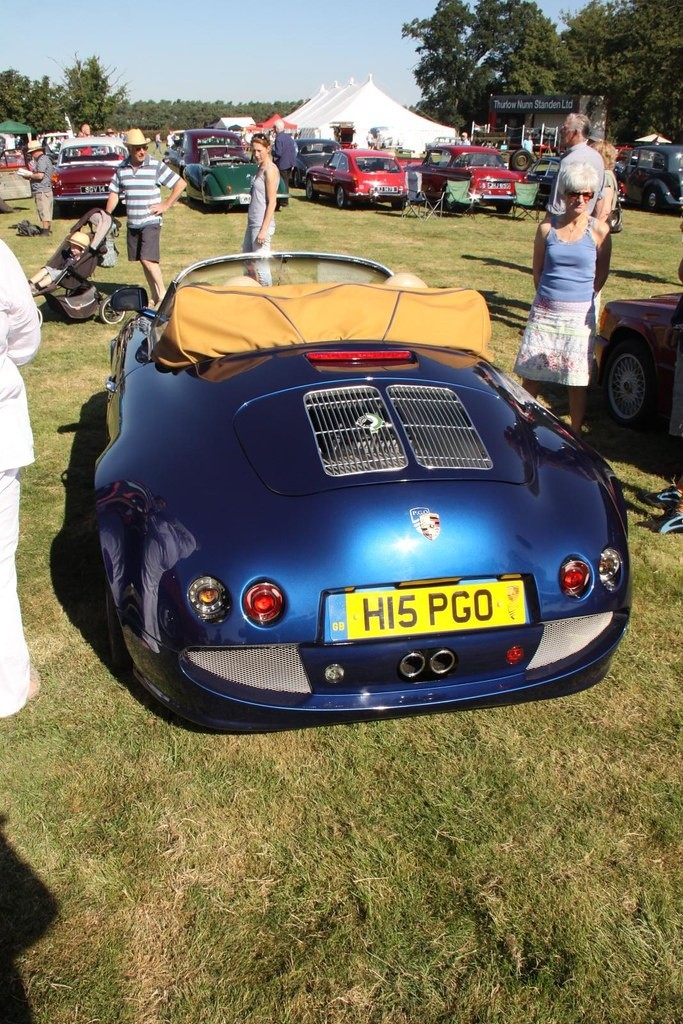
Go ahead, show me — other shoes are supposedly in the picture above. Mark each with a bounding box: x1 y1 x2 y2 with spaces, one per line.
153 299 161 312
29 283 39 294
148 299 155 309
38 229 49 236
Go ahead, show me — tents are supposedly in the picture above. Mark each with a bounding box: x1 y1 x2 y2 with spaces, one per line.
282 74 456 155
257 115 298 133
0 120 32 141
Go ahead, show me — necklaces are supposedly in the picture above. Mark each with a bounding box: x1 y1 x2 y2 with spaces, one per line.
567 224 575 233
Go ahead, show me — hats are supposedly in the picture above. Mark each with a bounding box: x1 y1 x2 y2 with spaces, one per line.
462 132 467 136
25 140 44 153
586 129 604 143
105 129 114 133
66 231 90 250
123 129 151 147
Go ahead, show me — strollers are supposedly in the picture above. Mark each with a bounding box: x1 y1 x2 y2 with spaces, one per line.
27 209 126 325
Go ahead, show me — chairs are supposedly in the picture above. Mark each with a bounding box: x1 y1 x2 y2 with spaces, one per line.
511 179 543 222
401 170 436 218
435 179 480 221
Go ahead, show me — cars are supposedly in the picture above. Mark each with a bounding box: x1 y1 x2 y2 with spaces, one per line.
0 133 130 217
412 146 524 216
526 156 628 235
289 139 408 210
164 128 294 208
616 145 683 214
90 251 683 733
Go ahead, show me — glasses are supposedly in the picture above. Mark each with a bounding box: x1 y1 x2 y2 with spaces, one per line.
254 134 268 144
565 189 594 201
129 146 148 151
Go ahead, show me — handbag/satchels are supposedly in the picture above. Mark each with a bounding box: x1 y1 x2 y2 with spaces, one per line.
604 207 623 233
100 223 119 268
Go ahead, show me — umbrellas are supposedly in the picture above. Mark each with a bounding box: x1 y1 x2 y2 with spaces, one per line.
635 134 671 143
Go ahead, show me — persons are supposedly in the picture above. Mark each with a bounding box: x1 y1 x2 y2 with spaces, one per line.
28 232 90 291
153 131 173 154
2 237 42 717
523 136 533 153
6 134 16 149
268 120 300 207
366 130 382 150
241 134 280 286
519 114 618 337
106 129 187 310
461 132 470 145
513 164 612 436
22 141 54 235
637 223 683 533
76 124 127 156
